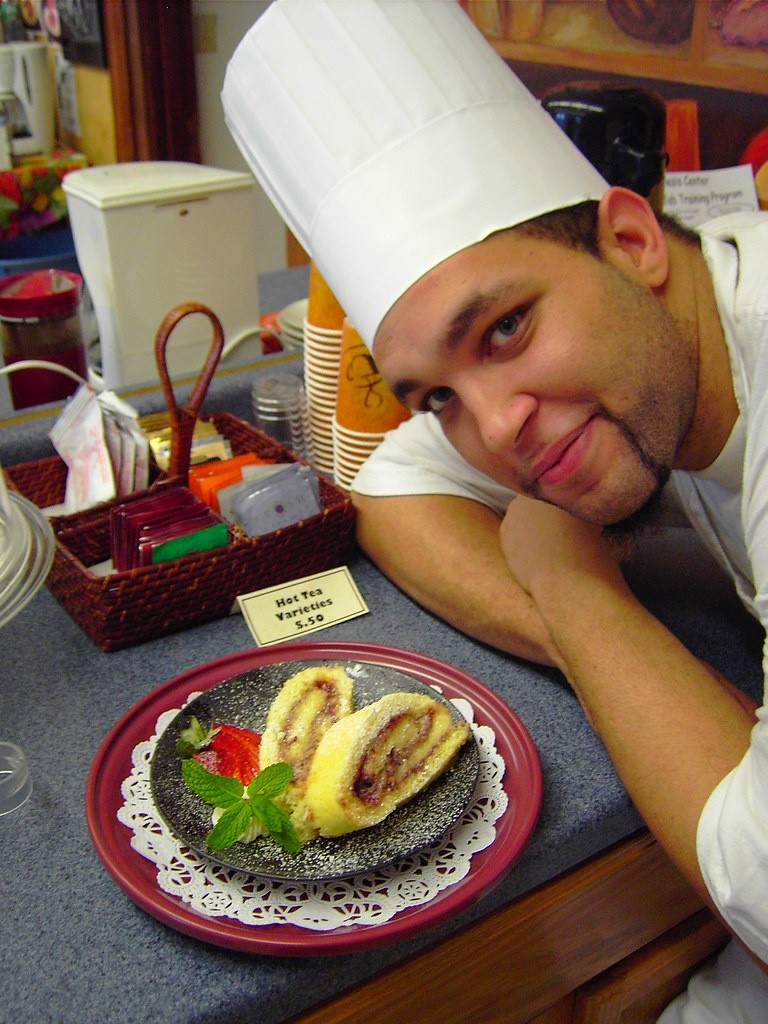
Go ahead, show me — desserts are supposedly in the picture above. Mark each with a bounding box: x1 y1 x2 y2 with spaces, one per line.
257 664 356 839
303 692 470 839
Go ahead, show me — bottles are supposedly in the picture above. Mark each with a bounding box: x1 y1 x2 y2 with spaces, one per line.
252 372 306 463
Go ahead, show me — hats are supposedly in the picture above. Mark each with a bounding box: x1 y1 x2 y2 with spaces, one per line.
219 0 610 356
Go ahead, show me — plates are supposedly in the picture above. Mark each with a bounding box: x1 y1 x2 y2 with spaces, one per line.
150 658 480 884
277 299 308 351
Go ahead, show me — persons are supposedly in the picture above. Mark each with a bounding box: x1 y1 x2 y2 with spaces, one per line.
218 0 767 1024
541 83 672 222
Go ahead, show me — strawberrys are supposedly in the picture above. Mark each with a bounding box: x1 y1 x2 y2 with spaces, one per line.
176 716 263 786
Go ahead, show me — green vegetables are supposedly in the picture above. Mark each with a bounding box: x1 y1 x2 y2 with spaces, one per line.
179 757 301 856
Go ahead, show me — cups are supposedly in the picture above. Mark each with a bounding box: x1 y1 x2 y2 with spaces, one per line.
0 269 88 412
302 257 412 495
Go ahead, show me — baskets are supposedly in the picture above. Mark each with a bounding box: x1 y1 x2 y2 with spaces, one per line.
4 412 352 654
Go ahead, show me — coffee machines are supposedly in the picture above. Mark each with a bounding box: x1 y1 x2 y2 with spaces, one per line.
0 40 63 155
